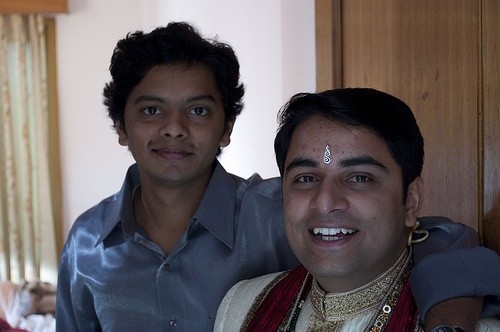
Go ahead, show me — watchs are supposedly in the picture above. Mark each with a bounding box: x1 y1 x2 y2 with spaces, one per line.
430 323 465 332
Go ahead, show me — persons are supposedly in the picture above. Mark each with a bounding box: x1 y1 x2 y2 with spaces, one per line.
56 22 500 332
214 86 500 332
